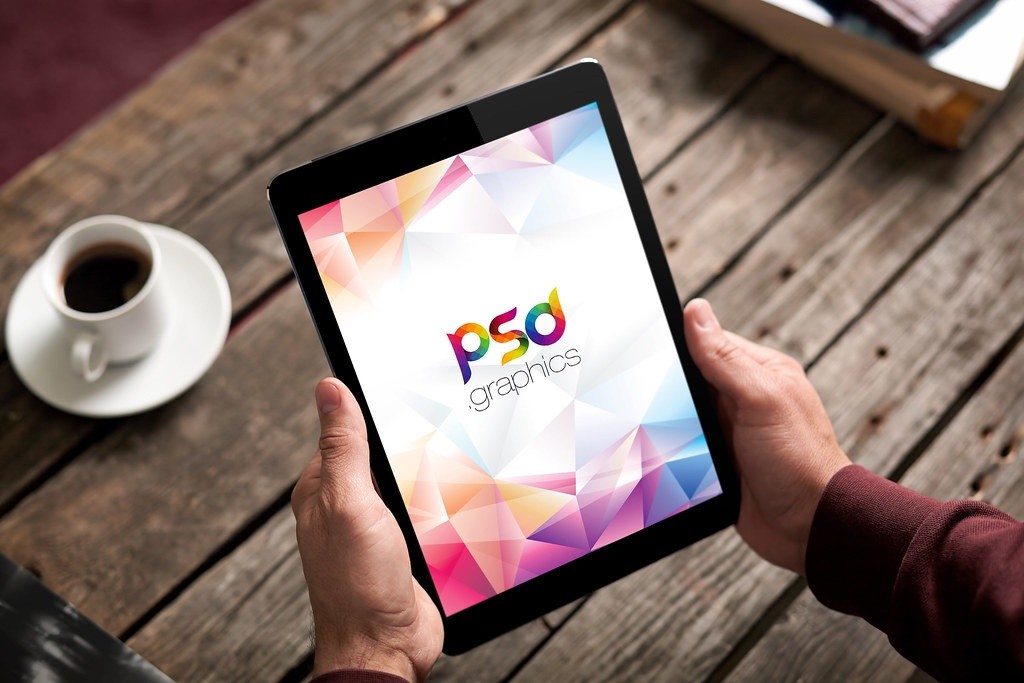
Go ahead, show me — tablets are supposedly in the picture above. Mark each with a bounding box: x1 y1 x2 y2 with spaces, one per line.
267 56 744 658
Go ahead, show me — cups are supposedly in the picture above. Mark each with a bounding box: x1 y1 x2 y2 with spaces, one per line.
41 214 164 383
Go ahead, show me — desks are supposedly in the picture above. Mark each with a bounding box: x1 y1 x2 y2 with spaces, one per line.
0 0 1024 683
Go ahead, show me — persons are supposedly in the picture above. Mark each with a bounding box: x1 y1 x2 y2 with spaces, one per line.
291 298 1024 683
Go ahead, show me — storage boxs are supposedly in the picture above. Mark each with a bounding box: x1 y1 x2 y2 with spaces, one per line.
707 0 1023 151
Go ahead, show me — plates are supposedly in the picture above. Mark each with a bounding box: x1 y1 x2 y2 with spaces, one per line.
5 221 232 418
